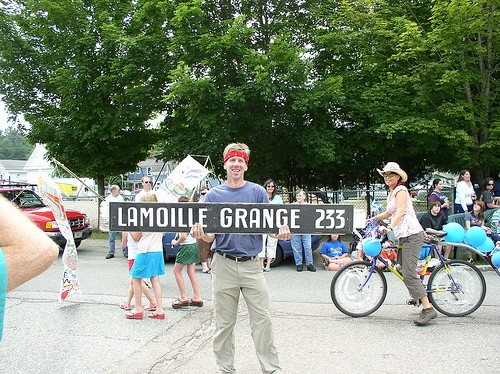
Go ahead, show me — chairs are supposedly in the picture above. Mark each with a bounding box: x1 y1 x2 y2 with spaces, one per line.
448 213 478 261
417 211 445 257
483 208 498 266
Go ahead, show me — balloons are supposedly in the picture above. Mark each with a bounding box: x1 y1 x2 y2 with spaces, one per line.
465 226 486 247
441 223 465 243
476 237 494 253
363 239 381 256
491 251 500 268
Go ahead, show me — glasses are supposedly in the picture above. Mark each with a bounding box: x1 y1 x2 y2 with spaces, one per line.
384 173 394 177
488 184 494 186
145 181 151 183
268 185 274 188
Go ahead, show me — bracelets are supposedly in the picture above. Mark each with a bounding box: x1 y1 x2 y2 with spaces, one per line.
389 227 393 231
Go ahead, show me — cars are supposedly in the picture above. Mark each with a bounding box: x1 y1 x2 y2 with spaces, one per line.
360 186 454 202
122 232 322 268
277 186 345 202
105 189 133 201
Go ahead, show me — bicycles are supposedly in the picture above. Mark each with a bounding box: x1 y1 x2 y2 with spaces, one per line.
329 217 487 317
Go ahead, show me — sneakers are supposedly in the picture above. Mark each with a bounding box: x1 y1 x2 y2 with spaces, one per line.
414 306 437 326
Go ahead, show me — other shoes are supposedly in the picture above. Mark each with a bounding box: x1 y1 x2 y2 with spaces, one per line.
120 303 131 309
297 265 303 271
125 312 143 319
263 268 271 272
148 313 166 319
172 298 190 309
307 264 316 272
146 305 156 310
189 299 203 306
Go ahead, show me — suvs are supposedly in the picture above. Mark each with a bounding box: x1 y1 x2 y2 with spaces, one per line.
0 174 92 251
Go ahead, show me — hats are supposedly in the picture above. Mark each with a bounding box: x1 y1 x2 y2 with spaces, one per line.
379 162 408 182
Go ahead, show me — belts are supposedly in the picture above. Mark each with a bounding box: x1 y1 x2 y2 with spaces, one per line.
216 249 256 263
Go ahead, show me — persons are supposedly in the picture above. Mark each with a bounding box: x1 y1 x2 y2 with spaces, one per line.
366 162 437 325
0 193 58 343
171 196 204 309
121 232 157 310
190 142 291 373
105 171 500 272
126 193 166 319
